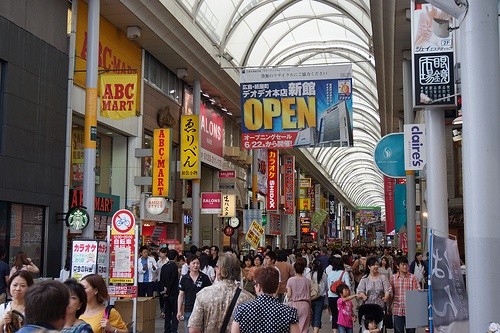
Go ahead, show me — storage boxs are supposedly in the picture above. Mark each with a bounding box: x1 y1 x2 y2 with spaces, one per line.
114 297 157 333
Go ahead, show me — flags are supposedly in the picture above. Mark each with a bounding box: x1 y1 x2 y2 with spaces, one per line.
100 74 138 119
428 235 470 327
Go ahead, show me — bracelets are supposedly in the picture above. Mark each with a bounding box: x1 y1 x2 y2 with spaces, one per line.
113 327 118 333
31 263 34 266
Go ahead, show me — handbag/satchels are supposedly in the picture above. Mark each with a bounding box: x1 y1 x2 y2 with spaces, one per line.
310 283 320 300
330 271 345 294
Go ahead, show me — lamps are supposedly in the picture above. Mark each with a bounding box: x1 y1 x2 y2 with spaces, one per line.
176 68 188 80
223 51 234 62
452 110 463 125
452 129 462 142
127 26 141 40
420 92 460 104
432 0 469 38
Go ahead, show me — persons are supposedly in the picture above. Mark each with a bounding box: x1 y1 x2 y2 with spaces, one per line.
239 238 430 333
0 251 128 333
231 267 300 333
459 254 465 275
138 244 218 333
187 252 255 333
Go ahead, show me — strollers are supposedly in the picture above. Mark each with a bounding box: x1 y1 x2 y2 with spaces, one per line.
358 295 387 333
